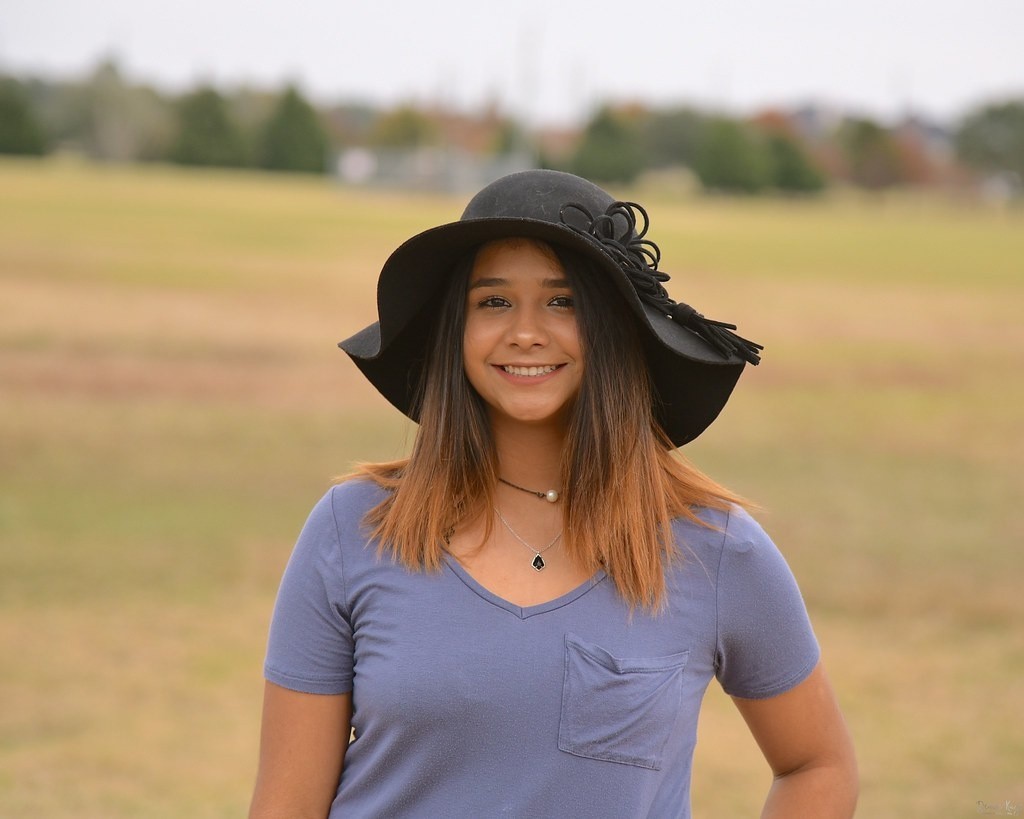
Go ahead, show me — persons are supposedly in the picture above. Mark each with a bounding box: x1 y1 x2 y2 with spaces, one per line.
248 170 859 819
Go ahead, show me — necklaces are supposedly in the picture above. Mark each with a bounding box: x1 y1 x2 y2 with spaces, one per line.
495 507 563 571
498 477 562 503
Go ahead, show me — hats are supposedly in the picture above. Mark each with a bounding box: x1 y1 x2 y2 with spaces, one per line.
337 168 764 453
